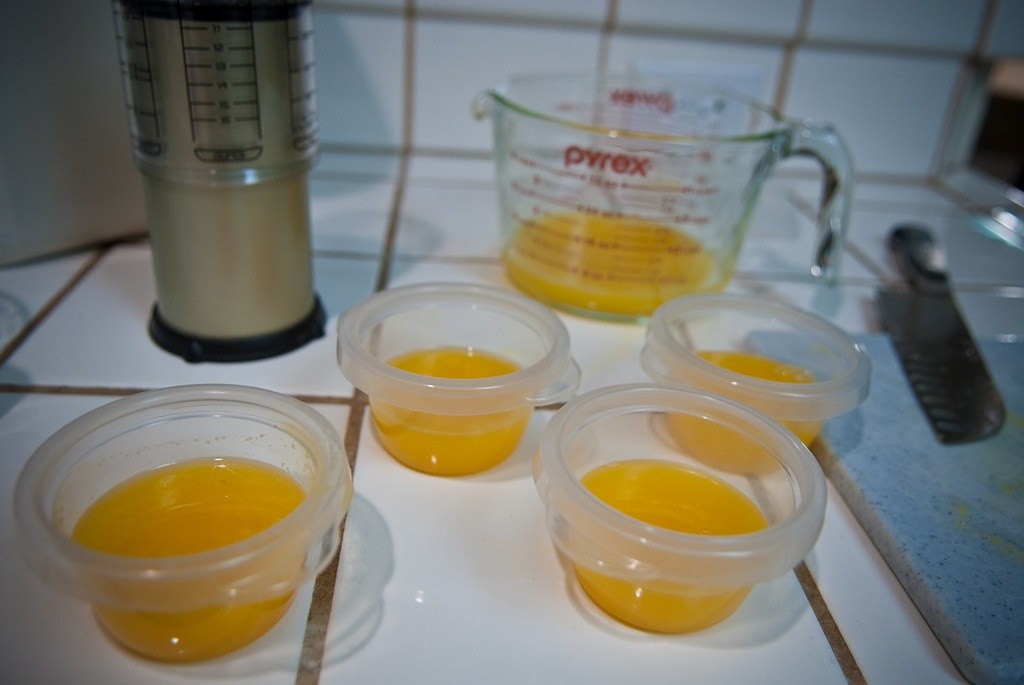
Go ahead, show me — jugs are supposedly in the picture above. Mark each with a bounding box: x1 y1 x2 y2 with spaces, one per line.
470 72 851 316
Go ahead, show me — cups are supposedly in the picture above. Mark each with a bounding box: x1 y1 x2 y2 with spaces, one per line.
14 385 351 664
335 281 580 479
534 385 828 634
639 291 877 471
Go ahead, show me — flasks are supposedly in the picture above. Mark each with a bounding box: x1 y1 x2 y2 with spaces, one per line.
114 2 327 362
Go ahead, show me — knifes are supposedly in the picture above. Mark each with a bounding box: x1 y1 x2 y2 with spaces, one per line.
868 217 1004 442
